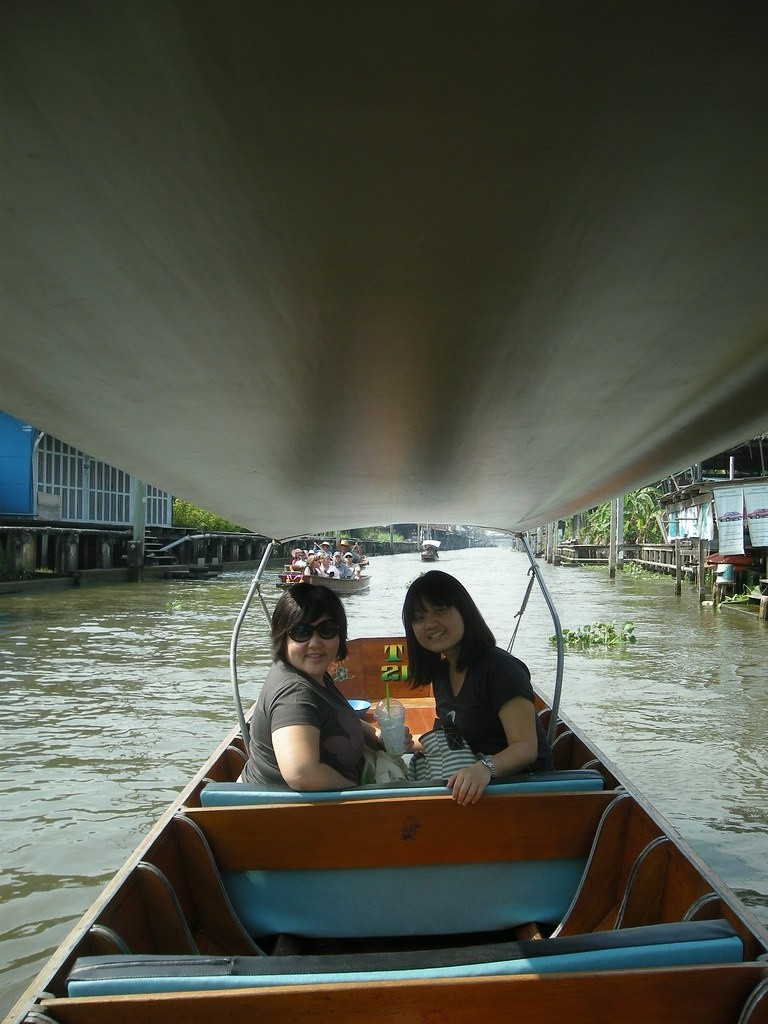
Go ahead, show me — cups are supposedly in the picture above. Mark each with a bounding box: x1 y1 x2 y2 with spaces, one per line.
373 697 406 756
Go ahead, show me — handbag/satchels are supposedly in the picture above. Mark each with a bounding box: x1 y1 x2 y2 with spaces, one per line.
361 741 408 784
408 711 478 783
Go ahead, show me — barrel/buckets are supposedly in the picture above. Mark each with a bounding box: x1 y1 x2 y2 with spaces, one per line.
716 564 735 583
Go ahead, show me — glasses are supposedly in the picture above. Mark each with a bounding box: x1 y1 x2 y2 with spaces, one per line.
345 556 352 559
287 620 342 642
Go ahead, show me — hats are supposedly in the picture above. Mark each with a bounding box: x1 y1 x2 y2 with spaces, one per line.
334 551 341 557
345 551 353 558
339 540 350 547
307 550 315 555
318 541 332 549
291 548 303 557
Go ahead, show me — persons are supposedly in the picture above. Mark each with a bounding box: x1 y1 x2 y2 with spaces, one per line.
235 582 414 791
401 570 538 806
291 540 361 580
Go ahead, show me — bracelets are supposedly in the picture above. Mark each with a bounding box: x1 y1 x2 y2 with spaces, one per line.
376 733 383 750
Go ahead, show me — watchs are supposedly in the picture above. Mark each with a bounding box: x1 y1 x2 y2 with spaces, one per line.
481 758 496 777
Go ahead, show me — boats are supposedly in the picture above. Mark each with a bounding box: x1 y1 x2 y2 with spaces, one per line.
420 539 441 562
275 560 372 595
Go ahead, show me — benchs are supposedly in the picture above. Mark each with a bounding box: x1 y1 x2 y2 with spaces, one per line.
67 917 746 997
197 768 605 941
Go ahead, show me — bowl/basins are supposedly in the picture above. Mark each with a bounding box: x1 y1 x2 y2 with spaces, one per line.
347 699 372 719
